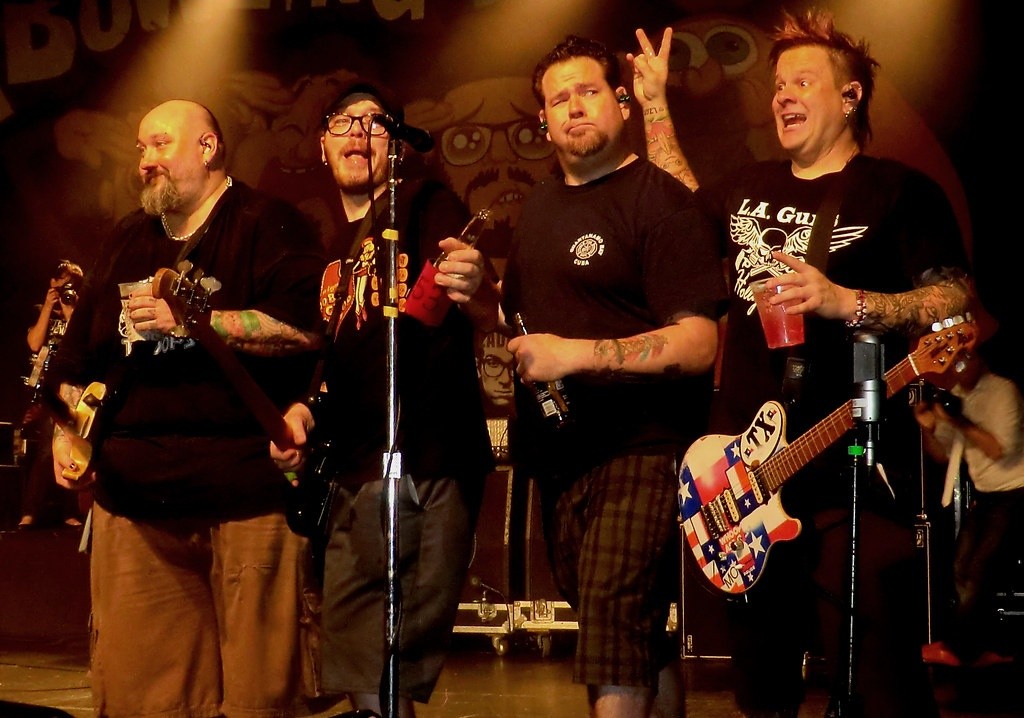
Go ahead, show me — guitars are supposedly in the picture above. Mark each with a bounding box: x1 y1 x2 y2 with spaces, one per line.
20 345 108 481
677 301 1000 595
152 259 350 592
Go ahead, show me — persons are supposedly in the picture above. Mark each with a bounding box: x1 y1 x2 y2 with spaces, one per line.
473 327 515 419
495 34 732 718
35 100 326 717
627 6 975 718
16 261 83 530
269 80 500 718
912 345 1024 658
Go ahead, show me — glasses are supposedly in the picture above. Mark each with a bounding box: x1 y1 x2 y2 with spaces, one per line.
325 112 387 136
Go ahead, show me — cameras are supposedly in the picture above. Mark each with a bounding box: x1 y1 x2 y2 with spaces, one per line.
922 385 947 402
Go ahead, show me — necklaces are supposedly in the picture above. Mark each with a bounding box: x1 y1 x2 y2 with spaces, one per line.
846 142 857 163
162 175 231 241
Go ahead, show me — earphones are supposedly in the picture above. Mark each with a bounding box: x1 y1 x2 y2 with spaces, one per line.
540 123 548 130
202 143 211 154
842 88 857 99
618 94 630 103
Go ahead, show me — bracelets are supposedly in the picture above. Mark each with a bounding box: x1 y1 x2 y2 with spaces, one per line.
845 290 867 329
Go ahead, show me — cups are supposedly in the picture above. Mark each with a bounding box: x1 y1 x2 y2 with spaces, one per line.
117 281 162 342
749 276 805 349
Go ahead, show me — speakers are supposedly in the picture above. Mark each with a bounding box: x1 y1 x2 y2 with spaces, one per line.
914 521 935 664
447 466 513 609
523 478 571 605
679 525 736 659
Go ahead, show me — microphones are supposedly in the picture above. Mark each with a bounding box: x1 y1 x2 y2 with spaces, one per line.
373 114 435 153
853 335 884 470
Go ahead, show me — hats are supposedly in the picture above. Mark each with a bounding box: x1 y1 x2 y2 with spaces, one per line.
322 79 434 153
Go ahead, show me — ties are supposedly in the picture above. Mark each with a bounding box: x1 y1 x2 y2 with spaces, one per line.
941 395 972 507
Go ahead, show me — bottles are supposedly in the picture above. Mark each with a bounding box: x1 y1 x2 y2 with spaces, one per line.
514 312 573 424
404 209 494 327
